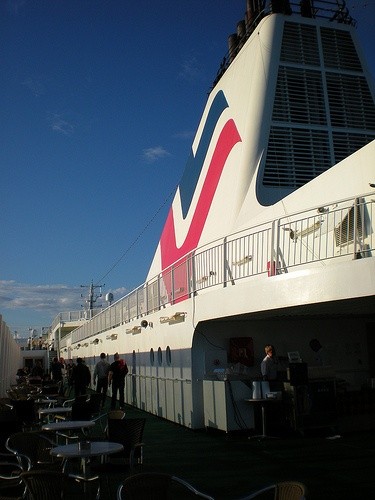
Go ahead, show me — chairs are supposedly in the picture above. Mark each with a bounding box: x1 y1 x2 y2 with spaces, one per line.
0 385 214 500
245 481 305 500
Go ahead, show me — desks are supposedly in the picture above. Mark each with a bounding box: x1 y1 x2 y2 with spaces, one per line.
50 442 124 493
37 408 72 424
35 400 57 409
242 398 277 439
42 421 94 445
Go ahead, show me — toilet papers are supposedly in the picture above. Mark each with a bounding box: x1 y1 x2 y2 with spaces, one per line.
262 381 270 398
252 381 261 400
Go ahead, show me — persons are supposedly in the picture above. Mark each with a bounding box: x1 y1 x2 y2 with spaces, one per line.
261 344 280 375
108 353 129 411
49 357 67 384
68 357 90 400
38 336 43 349
92 352 112 408
49 339 54 351
32 359 46 377
27 336 36 350
